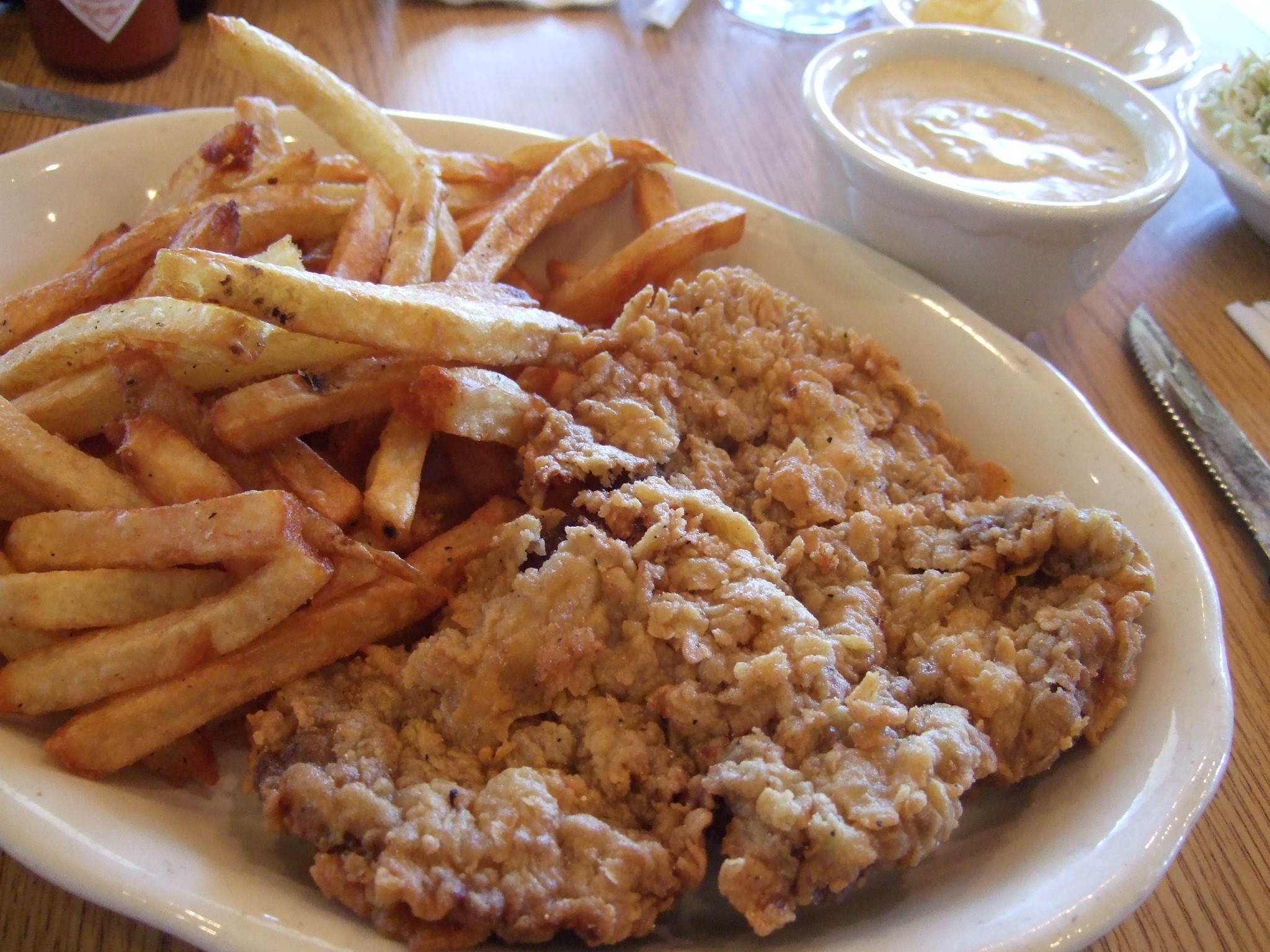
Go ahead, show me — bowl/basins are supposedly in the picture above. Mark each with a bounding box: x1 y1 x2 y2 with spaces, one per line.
871 0 1203 94
803 23 1191 341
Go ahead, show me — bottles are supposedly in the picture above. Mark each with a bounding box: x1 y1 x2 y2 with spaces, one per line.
29 1 180 84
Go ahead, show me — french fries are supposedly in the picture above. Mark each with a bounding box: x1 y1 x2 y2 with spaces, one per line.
1 9 747 778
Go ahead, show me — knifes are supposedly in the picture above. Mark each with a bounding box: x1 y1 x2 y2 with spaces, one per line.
1130 303 1269 562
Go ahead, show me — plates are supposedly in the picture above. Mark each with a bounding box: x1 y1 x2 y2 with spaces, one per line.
0 108 1233 952
